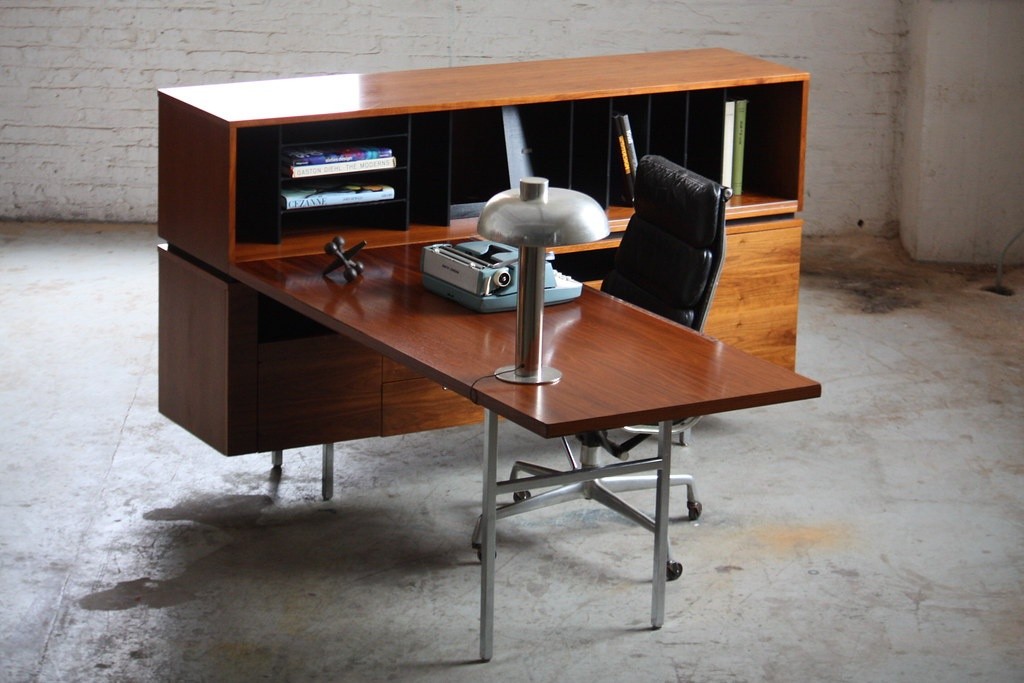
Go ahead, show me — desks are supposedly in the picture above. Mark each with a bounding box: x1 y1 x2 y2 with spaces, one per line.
232 237 822 662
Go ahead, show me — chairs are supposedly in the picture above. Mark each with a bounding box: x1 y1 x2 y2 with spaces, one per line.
472 156 734 578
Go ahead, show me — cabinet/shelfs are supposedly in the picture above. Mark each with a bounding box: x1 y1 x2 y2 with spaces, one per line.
156 45 810 500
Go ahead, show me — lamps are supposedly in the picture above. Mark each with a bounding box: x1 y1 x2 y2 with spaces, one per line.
477 177 611 386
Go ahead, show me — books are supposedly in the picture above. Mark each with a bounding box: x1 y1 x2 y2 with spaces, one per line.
277 148 396 179
281 184 394 209
613 113 637 204
721 98 747 195
